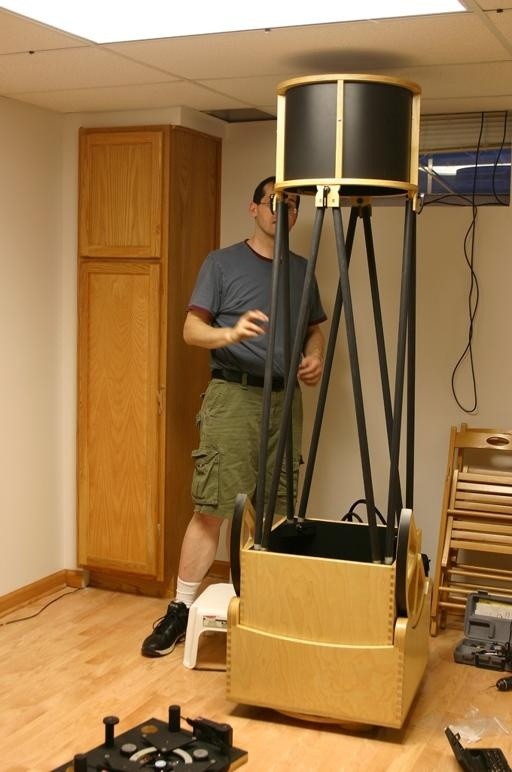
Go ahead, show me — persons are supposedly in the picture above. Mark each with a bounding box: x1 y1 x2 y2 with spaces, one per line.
141 174 328 658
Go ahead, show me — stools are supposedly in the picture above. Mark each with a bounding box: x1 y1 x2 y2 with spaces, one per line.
183 580 238 669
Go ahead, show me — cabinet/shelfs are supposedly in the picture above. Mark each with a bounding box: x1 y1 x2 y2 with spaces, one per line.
74 126 219 591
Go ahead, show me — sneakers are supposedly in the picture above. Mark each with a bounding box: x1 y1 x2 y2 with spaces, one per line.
141 601 189 656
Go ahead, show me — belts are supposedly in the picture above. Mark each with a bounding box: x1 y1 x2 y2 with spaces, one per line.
212 368 286 393
